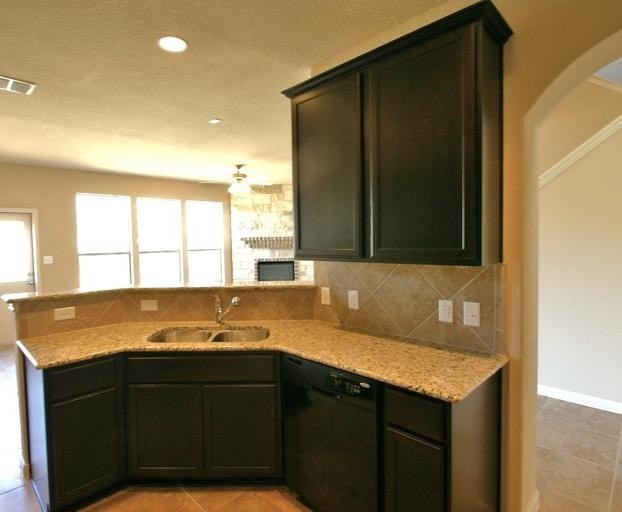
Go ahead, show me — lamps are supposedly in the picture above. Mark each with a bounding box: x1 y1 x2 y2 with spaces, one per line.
228 181 252 195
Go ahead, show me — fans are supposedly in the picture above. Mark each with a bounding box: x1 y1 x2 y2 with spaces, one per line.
199 164 274 187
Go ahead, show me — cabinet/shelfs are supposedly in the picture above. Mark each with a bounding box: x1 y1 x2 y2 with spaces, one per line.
280 1 514 267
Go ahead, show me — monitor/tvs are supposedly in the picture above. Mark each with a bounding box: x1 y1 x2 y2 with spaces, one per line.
257 261 294 281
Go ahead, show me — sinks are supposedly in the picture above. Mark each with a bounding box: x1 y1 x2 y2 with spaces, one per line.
147 325 213 344
213 325 269 342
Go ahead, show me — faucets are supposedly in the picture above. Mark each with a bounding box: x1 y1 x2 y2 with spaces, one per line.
214 294 241 321
